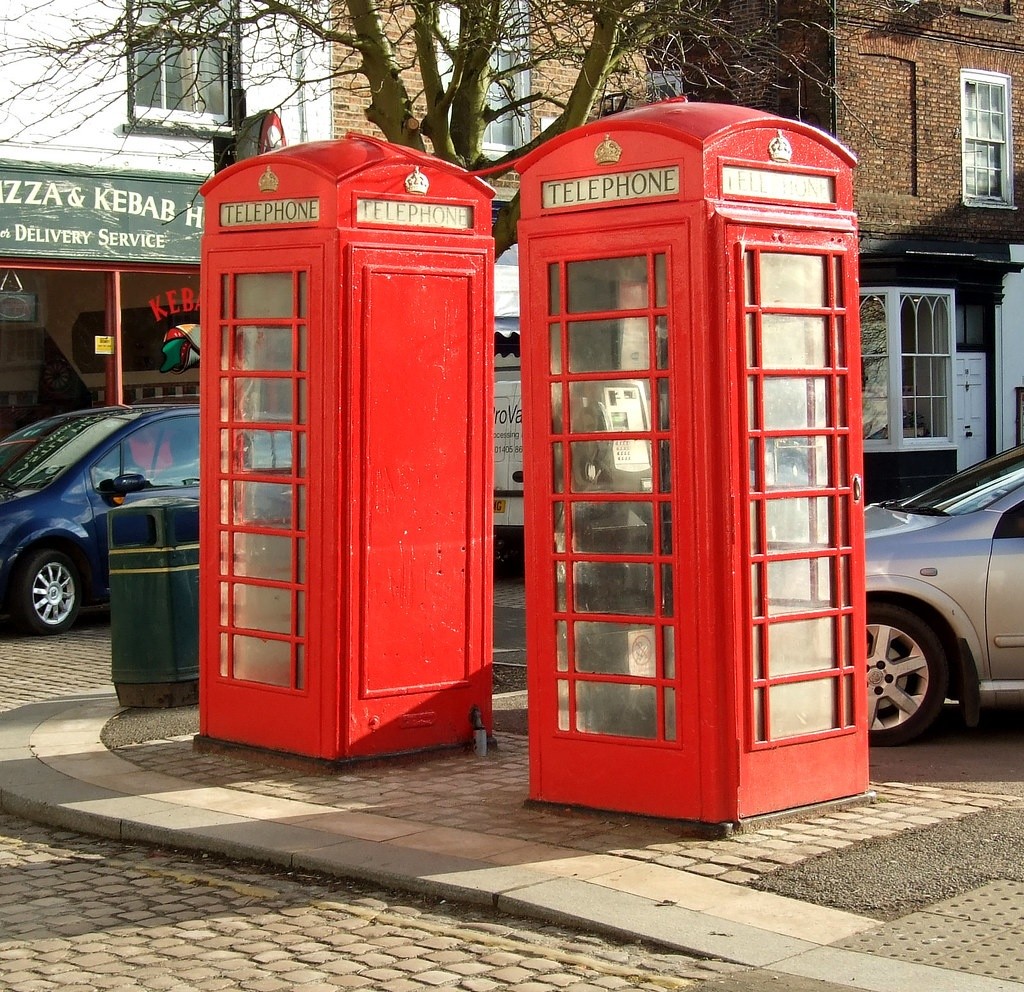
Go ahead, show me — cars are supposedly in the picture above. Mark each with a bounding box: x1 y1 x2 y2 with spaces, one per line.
1 403 199 633
862 443 1023 746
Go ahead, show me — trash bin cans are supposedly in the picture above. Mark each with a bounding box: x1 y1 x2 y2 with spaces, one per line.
105 495 200 709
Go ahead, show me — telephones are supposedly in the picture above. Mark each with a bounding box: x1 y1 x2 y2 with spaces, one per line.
564 372 658 504
